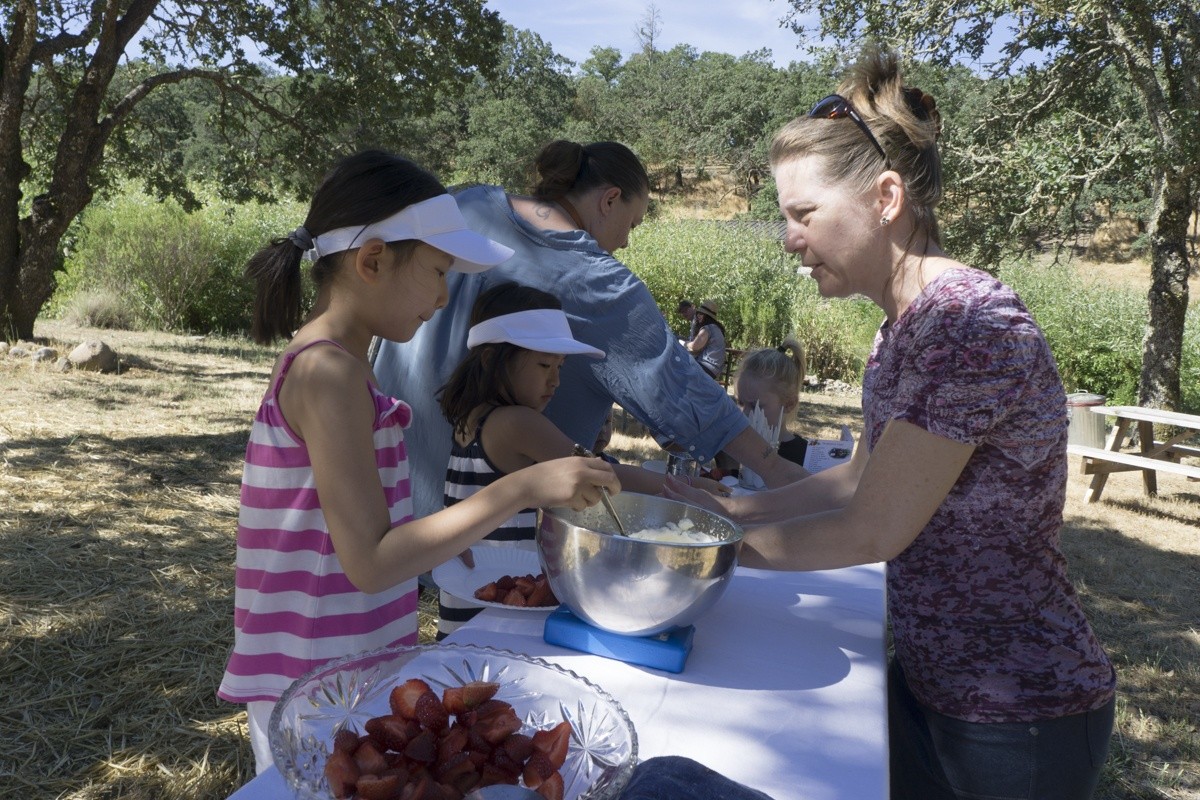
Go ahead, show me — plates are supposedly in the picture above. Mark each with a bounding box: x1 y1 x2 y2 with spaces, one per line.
432 546 560 611
642 460 739 486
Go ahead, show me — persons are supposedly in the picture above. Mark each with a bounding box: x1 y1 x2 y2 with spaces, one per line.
648 339 808 479
216 149 621 776
431 282 607 642
678 300 699 342
678 299 726 379
366 139 813 600
659 55 1116 800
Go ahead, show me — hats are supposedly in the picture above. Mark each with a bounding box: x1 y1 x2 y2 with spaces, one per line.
676 301 691 313
467 308 606 358
696 302 718 321
308 194 515 273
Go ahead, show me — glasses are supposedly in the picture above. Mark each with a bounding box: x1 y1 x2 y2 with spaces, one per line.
810 95 891 170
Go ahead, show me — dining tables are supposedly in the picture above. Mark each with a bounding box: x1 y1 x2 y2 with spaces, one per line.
226 441 890 800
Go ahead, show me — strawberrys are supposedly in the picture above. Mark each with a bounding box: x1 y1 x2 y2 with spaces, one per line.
700 466 722 482
474 572 559 606
326 680 572 799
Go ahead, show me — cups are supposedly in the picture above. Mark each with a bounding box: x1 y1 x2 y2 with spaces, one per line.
666 453 697 488
739 441 780 490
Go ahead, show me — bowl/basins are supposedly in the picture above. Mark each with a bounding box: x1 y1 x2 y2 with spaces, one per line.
267 642 639 800
535 491 744 637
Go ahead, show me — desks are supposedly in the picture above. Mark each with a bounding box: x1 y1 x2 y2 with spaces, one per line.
1085 406 1200 507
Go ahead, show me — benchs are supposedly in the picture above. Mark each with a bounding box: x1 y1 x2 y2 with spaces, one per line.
1066 439 1200 488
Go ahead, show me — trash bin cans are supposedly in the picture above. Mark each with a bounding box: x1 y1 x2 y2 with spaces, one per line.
1066 393 1107 449
